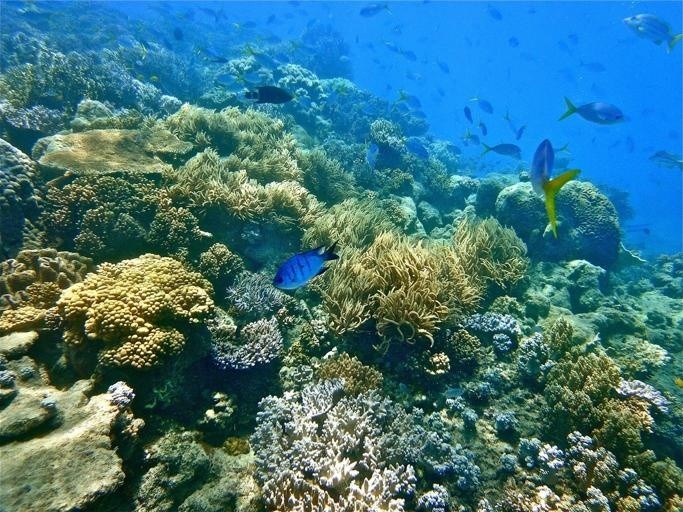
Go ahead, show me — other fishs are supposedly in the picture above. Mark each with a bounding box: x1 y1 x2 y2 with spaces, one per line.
140 1 450 120
